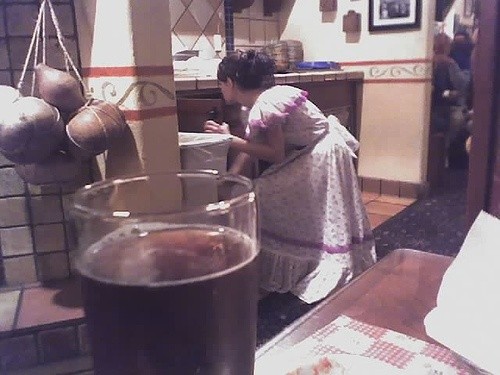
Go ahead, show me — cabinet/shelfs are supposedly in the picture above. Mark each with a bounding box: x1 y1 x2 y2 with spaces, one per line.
174 82 359 209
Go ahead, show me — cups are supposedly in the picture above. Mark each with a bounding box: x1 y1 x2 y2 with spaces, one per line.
71 169 260 375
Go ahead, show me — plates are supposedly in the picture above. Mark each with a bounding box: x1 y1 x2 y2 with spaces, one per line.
296 61 344 73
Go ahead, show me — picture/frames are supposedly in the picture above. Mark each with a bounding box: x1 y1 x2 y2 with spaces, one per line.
367 0 421 33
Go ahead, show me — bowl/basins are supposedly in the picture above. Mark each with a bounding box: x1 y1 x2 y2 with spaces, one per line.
261 39 304 75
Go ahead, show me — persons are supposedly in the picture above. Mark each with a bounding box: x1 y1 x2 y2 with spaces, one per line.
204 48 379 305
434 25 480 171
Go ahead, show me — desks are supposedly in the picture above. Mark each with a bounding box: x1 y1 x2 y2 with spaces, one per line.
253 248 499 375
